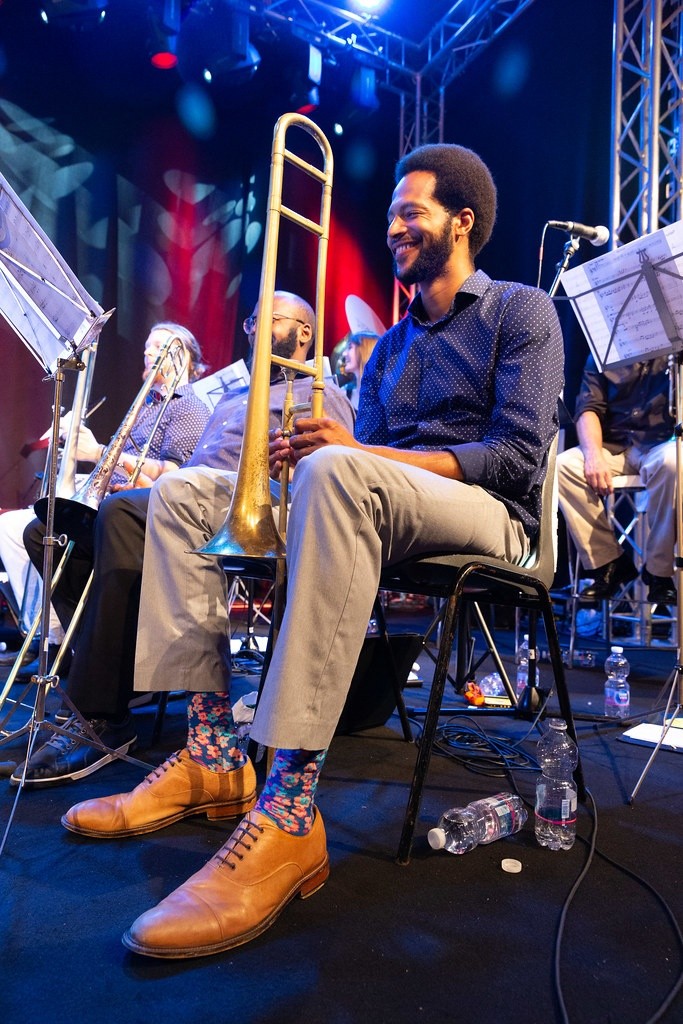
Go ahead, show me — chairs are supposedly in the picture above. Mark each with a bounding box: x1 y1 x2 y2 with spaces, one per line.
373 432 587 865
152 555 287 766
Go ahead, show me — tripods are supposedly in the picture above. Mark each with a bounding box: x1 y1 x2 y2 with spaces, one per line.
404 233 683 807
0 184 164 854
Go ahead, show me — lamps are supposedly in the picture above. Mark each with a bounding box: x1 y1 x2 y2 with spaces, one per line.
41 1 379 135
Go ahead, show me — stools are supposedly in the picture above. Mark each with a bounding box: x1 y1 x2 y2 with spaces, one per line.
568 474 677 669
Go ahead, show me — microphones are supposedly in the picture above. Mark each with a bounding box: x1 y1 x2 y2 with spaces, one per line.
549 220 609 246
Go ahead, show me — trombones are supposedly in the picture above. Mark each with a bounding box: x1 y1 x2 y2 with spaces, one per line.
35 335 99 534
0 331 189 737
181 110 339 780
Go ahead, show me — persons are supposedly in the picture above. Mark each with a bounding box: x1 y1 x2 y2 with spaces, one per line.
340 329 380 415
9 291 356 789
557 352 677 606
0 323 212 684
61 145 565 958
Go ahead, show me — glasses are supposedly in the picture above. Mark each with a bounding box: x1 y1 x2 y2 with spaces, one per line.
242 312 306 335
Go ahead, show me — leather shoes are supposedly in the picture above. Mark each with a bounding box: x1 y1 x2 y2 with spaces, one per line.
580 552 639 602
60 746 259 839
641 562 677 602
122 801 330 959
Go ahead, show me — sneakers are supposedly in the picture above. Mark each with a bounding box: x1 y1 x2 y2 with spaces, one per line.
54 685 160 725
9 712 141 790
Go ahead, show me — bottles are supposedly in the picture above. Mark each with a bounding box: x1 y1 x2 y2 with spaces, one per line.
516 634 539 700
534 718 578 850
427 792 528 855
604 647 630 719
479 672 505 697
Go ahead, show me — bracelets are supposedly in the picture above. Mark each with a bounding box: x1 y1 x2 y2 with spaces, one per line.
95 444 105 465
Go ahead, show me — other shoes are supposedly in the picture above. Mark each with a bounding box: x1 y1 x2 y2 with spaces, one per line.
15 648 74 683
0 645 41 666
